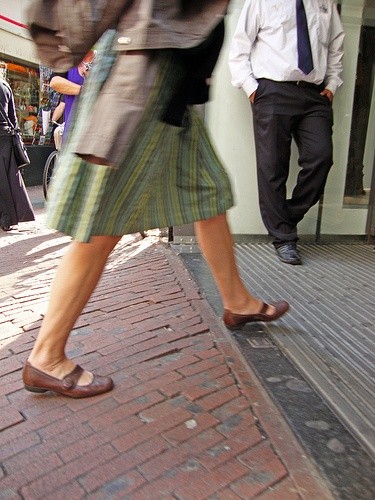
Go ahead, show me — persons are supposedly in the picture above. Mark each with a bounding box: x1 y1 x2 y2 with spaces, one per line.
49 94 66 145
22 0 289 399
49 49 99 151
228 0 345 265
0 78 35 231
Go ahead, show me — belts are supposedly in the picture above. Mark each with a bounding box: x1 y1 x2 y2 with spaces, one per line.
284 78 321 91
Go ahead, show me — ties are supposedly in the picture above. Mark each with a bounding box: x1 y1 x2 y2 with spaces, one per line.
294 0 314 76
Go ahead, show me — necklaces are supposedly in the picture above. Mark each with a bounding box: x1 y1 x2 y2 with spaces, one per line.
80 55 97 71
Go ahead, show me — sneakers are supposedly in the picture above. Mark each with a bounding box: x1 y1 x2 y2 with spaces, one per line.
276 243 303 267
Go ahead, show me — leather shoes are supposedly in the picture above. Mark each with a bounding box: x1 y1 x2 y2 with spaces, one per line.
223 297 291 330
22 358 114 400
